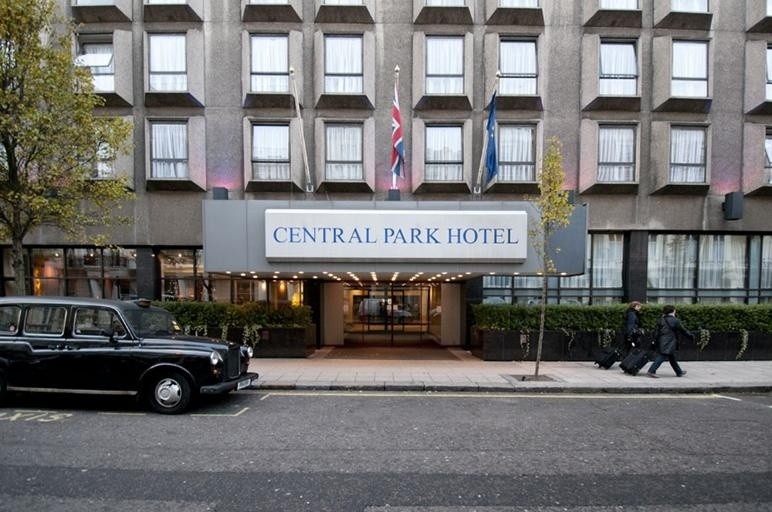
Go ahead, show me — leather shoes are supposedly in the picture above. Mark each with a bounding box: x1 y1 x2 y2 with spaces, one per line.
676 370 687 377
647 372 660 378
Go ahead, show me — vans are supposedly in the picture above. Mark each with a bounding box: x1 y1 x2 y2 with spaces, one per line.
358 298 412 324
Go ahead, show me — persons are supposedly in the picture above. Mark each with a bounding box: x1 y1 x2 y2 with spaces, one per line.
624 302 642 355
647 305 696 380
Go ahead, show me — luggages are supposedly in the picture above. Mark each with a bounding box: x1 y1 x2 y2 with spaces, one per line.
594 345 620 369
619 342 655 376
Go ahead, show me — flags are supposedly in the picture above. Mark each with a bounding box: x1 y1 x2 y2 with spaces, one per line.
486 88 499 186
388 76 407 181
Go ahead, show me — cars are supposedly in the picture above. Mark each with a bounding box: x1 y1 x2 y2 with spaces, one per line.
0 296 259 415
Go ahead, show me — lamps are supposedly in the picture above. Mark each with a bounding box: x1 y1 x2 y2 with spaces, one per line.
721 191 744 220
212 186 228 202
388 189 400 201
